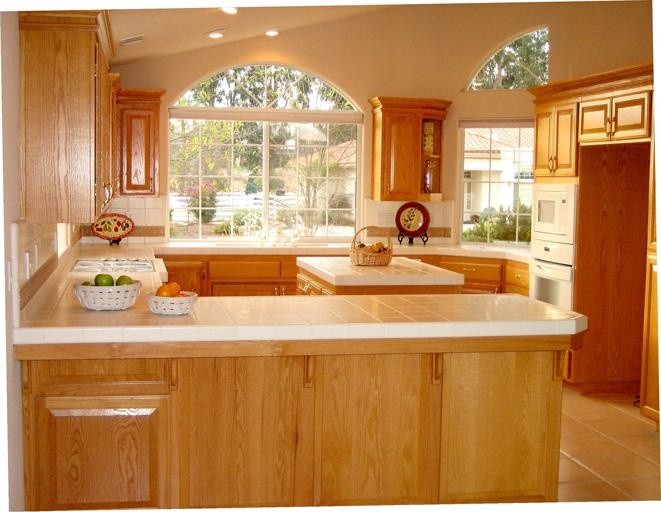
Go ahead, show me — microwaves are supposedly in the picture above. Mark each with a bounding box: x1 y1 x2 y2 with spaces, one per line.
529 182 575 245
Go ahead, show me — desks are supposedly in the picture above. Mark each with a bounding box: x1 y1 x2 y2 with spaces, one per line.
293 254 464 297
13 291 591 512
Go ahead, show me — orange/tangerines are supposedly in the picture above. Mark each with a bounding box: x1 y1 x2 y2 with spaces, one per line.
81 274 133 286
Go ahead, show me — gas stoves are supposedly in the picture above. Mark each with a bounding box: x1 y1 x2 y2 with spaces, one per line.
68 257 155 275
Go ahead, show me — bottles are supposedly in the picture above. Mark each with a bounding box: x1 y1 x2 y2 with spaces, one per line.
422 160 433 194
422 120 435 156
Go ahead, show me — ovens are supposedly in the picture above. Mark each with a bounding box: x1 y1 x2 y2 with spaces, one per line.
527 257 573 313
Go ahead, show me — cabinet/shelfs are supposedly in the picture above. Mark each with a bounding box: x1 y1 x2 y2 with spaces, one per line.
117 85 167 199
437 255 501 295
531 181 647 267
391 254 437 266
153 254 206 297
575 61 653 146
17 11 120 226
368 96 456 203
501 257 530 296
525 80 650 186
206 254 350 296
637 141 661 423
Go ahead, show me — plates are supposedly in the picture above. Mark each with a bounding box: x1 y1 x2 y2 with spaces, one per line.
394 202 430 238
90 212 135 241
